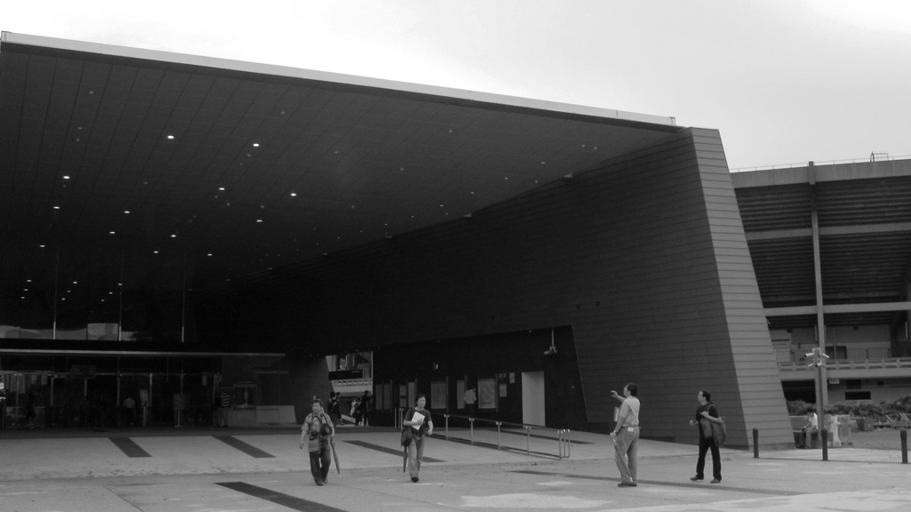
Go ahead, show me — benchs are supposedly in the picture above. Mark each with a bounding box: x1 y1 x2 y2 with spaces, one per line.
791 415 858 447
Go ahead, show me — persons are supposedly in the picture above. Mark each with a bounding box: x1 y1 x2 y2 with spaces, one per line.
687 389 726 484
795 406 820 448
609 382 643 487
463 385 478 428
401 393 434 482
330 389 374 426
298 398 338 486
221 389 233 428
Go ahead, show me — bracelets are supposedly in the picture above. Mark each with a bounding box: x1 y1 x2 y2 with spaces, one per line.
613 428 618 434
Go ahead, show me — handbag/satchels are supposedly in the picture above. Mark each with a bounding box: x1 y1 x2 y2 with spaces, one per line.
401 428 412 447
308 421 332 453
700 418 713 439
712 424 727 445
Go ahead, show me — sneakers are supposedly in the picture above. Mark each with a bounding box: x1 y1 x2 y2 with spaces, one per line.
315 478 328 486
618 480 638 486
711 477 721 483
411 475 421 482
691 474 704 481
